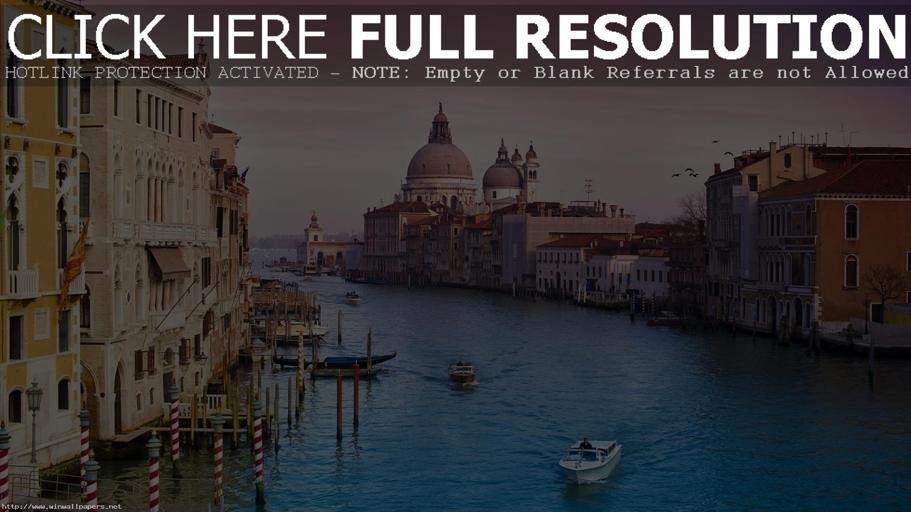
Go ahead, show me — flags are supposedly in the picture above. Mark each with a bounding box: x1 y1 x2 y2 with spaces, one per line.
58 219 91 310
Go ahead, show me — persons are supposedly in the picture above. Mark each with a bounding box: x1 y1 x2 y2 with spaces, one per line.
580 438 592 458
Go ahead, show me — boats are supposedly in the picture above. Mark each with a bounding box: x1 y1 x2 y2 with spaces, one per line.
559 439 623 487
273 352 399 377
447 362 475 388
346 294 360 307
